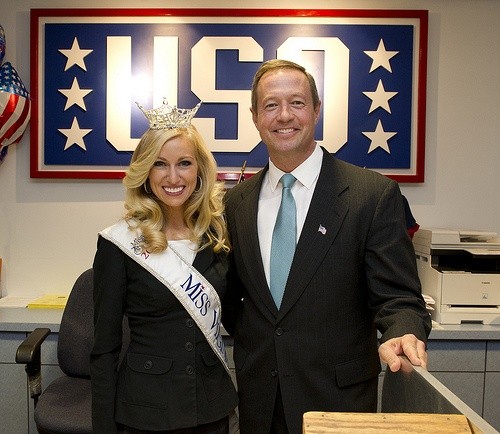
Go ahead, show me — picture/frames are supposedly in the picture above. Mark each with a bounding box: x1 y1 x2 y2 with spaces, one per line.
30 8 428 182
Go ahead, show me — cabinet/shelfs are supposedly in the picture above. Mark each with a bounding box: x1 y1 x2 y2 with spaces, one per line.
0 305 500 434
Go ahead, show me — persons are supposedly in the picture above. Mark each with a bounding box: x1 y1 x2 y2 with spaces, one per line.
70 114 248 434
205 58 431 433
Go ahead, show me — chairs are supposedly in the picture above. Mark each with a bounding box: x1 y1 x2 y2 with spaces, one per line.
15 269 101 433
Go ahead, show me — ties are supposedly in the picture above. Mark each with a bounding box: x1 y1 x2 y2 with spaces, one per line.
270 173 297 311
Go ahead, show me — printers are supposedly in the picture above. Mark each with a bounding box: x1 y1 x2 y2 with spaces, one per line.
413 228 500 326
